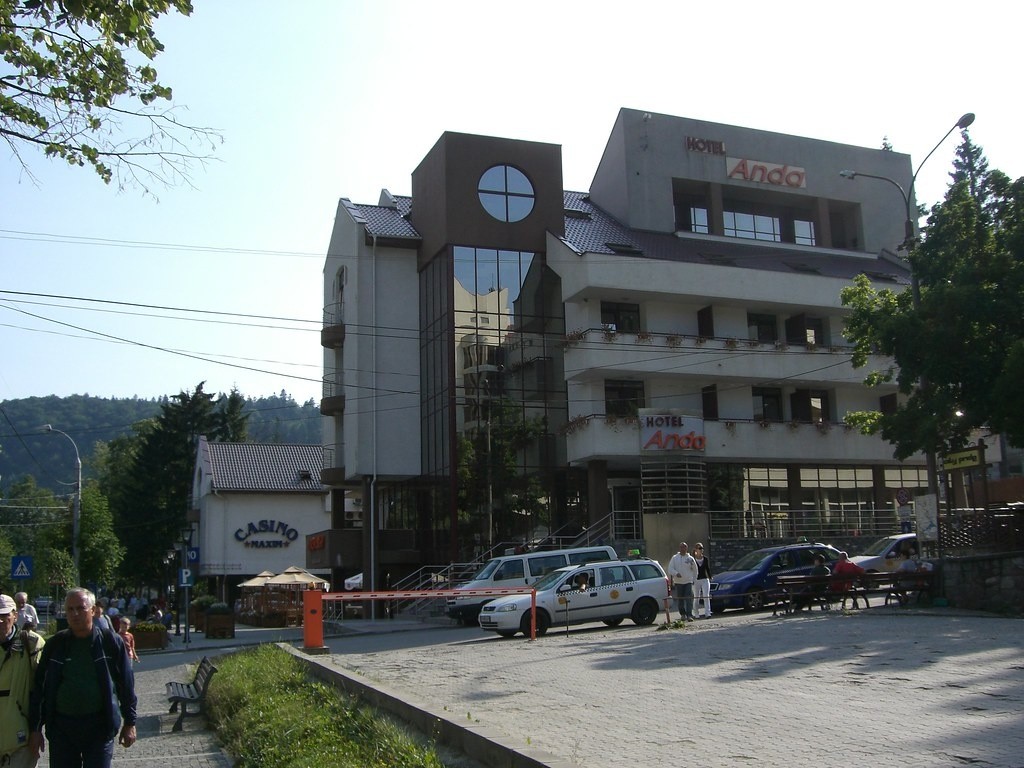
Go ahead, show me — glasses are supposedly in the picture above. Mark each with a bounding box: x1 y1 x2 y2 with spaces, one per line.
696 547 704 550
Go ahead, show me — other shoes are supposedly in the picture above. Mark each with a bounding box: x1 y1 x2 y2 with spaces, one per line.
695 615 700 619
686 616 694 622
681 614 688 621
706 615 712 619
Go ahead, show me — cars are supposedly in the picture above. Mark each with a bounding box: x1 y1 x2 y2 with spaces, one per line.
847 534 932 591
707 536 848 617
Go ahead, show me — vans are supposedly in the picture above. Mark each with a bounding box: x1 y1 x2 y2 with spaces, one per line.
444 545 623 625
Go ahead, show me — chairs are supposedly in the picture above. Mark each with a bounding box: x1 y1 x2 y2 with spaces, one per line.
588 577 595 587
572 575 580 590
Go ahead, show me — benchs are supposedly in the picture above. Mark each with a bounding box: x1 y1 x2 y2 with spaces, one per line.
770 567 940 616
165 655 218 733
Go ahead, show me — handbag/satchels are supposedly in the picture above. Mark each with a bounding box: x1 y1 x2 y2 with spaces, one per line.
911 560 925 586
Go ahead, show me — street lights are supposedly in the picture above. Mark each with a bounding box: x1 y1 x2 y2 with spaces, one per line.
182 524 193 643
35 422 82 590
839 113 977 512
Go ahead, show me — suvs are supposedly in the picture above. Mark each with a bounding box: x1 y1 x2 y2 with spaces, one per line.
34 596 56 616
476 556 670 639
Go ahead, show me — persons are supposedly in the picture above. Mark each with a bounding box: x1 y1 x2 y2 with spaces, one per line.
27 588 139 768
823 552 865 610
0 593 46 767
690 542 713 619
13 588 173 670
893 550 927 606
668 542 699 622
572 573 590 591
793 554 830 614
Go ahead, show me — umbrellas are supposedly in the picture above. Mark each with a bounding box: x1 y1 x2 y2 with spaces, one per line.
237 570 276 614
264 566 330 627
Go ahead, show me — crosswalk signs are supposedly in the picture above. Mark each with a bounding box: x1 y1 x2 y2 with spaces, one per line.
11 555 33 581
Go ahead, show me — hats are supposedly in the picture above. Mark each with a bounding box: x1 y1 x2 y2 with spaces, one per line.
0 594 16 614
95 601 104 608
110 598 117 604
